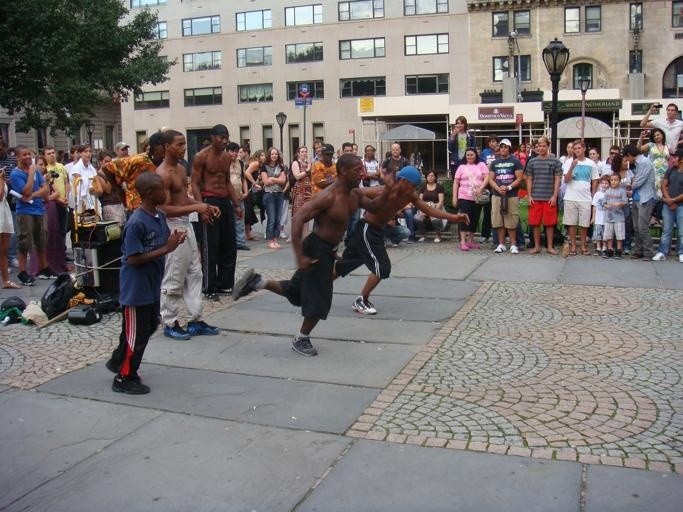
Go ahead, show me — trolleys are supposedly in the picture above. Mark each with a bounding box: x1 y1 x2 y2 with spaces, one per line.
70 176 99 290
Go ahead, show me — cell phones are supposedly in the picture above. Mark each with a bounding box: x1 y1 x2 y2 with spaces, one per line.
454 126 458 130
573 156 576 160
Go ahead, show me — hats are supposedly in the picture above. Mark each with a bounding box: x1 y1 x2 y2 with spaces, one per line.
499 138 511 147
115 141 131 150
667 148 683 159
396 165 424 185
320 142 335 156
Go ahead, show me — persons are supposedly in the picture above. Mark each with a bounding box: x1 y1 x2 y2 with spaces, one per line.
230 151 399 355
150 131 221 341
189 123 246 304
639 103 683 151
108 172 186 396
446 116 474 183
320 166 470 318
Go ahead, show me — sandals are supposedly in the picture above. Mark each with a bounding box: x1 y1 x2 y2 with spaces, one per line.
2 279 22 290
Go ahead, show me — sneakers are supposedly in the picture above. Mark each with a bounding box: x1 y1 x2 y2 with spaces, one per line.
185 320 220 337
231 267 261 301
291 334 319 358
351 294 377 316
387 234 683 266
234 228 293 251
205 292 222 302
17 266 60 286
104 358 124 373
163 317 191 342
110 373 153 395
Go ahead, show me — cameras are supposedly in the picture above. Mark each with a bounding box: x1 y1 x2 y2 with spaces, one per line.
47 170 59 178
656 105 662 108
644 130 651 134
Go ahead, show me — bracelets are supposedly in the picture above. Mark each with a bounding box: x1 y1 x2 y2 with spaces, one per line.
232 203 241 209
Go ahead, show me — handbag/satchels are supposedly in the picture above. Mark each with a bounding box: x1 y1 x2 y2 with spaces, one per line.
0 273 76 324
472 185 491 205
651 200 663 219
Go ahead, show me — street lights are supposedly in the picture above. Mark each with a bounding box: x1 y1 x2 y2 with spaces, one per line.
275 111 287 152
509 32 523 147
539 38 570 156
85 121 94 165
578 74 590 145
